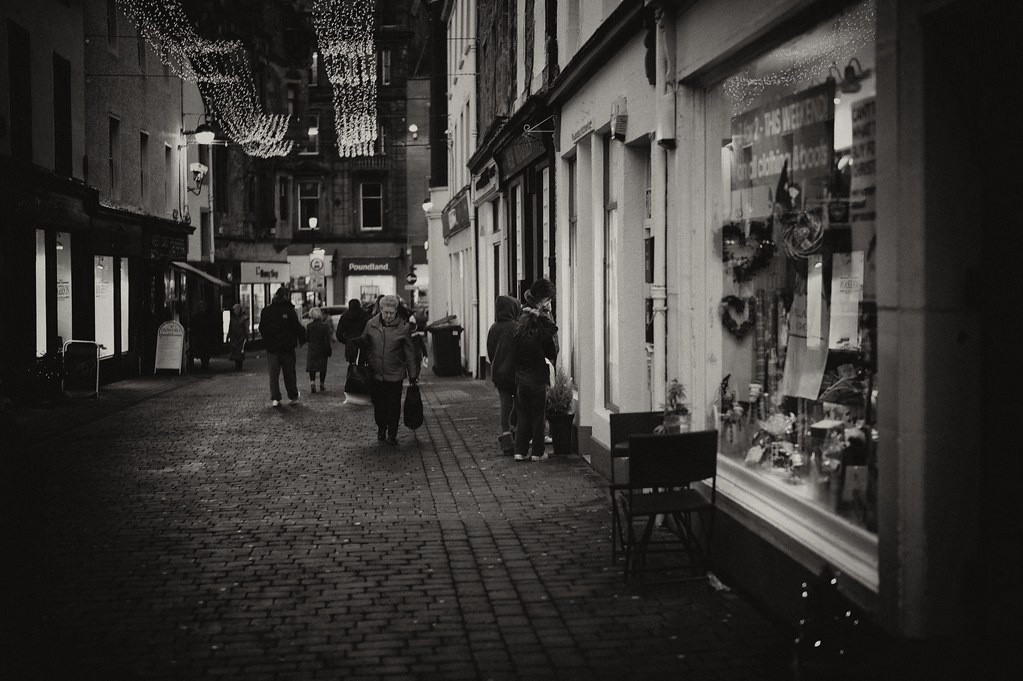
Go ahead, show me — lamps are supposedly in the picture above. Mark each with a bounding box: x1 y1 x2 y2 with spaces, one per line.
820 56 869 102
194 115 216 144
188 162 208 197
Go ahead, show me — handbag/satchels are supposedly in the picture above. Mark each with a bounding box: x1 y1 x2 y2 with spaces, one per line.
343 345 375 406
510 406 518 426
404 382 424 431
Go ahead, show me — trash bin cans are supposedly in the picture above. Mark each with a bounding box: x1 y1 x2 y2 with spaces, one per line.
424 314 467 378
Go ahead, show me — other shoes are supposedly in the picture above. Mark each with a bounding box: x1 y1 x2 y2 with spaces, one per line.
377 429 387 441
320 388 326 390
386 437 398 445
288 390 301 405
311 382 317 393
529 435 553 444
531 452 548 461
272 399 279 407
514 454 529 461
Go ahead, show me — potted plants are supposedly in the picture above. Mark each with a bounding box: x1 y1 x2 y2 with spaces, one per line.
22 363 69 406
546 373 576 455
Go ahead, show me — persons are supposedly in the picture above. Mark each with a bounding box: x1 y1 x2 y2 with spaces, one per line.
487 278 558 461
188 285 428 446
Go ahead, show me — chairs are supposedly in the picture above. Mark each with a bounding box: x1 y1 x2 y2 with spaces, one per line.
607 411 720 584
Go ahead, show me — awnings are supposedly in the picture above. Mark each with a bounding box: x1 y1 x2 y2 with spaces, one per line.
173 261 232 295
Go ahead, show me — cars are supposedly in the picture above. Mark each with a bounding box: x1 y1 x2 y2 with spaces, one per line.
302 304 350 337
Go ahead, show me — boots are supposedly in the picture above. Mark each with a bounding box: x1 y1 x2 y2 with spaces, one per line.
498 429 517 456
